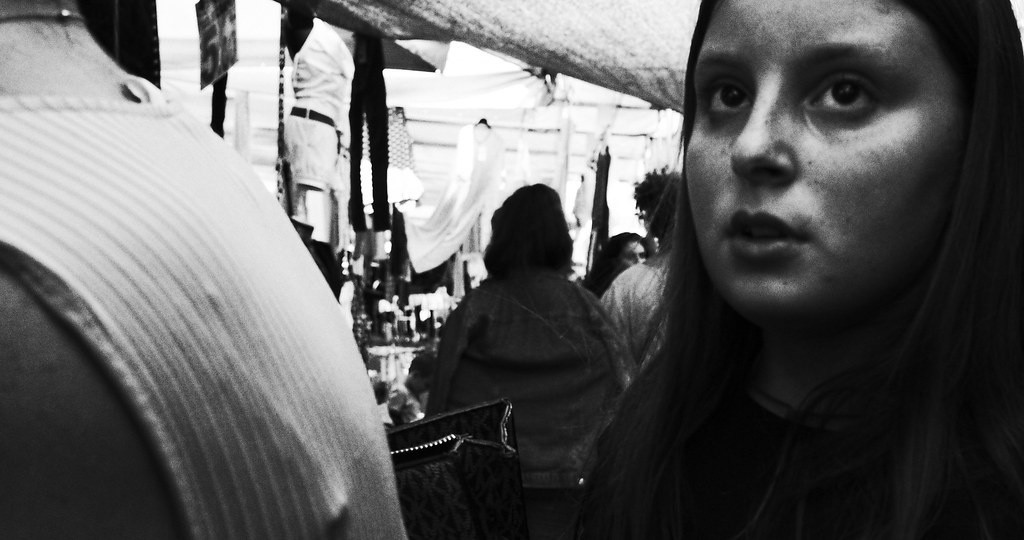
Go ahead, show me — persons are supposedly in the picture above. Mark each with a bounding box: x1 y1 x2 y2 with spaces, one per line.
567 0 1024 539
0 0 409 540
425 167 683 540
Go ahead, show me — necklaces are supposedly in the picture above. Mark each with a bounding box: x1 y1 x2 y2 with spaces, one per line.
0 9 86 25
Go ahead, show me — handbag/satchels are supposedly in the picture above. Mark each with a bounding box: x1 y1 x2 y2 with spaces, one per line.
386 398 530 540
